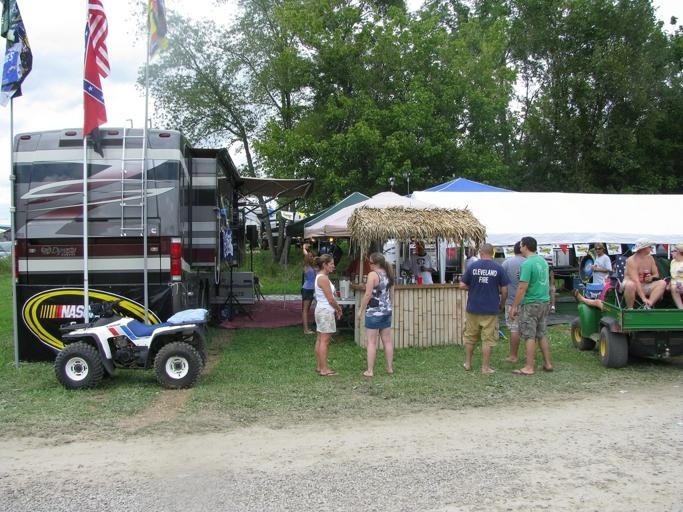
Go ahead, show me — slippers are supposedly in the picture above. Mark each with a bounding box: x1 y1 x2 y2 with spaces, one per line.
364 371 373 376
463 363 471 370
512 368 535 375
317 367 320 371
320 370 334 376
482 369 496 374
543 364 553 372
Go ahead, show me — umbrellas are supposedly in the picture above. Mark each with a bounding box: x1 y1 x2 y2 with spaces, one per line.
277 207 317 223
303 191 442 239
414 175 517 195
284 206 334 253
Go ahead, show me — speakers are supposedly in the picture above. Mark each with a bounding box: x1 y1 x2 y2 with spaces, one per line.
246 225 257 240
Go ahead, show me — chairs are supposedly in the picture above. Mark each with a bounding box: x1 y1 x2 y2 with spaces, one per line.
609 255 647 310
654 258 672 300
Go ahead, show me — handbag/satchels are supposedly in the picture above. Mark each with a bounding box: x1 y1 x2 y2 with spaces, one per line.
367 270 381 308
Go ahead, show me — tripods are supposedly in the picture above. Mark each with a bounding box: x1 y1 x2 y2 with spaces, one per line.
220 265 255 322
251 243 266 300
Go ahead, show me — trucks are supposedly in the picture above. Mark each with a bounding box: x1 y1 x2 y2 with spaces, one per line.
570 300 683 368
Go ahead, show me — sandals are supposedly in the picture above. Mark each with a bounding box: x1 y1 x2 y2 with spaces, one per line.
304 330 314 334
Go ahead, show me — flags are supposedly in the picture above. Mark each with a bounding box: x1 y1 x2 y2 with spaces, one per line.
82 0 111 160
0 0 33 107
145 0 169 60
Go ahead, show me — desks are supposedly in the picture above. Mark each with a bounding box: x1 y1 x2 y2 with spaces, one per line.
334 298 355 331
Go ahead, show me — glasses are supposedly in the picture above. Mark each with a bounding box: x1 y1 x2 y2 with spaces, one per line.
672 250 677 252
595 247 602 250
369 261 376 264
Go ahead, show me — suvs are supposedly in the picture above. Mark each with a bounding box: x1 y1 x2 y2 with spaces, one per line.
54 299 208 389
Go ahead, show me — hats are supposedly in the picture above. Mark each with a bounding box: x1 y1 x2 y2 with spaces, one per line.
632 239 652 252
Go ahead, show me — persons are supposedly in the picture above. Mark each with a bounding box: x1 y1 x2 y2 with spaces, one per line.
544 255 556 314
464 240 479 275
301 243 316 335
500 241 526 364
357 252 395 377
458 243 511 375
590 243 613 285
571 261 617 310
557 244 574 290
493 247 507 266
312 253 341 375
404 241 437 283
509 237 553 375
667 243 683 310
619 238 667 310
